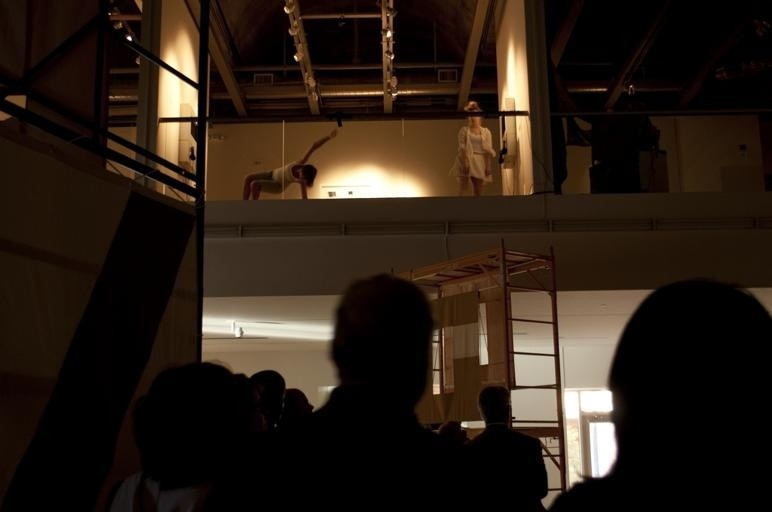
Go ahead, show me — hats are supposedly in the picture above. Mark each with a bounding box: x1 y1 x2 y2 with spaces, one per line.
465 101 480 112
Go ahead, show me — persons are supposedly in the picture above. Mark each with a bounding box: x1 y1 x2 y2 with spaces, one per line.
109 273 772 512
455 101 497 197
242 129 337 200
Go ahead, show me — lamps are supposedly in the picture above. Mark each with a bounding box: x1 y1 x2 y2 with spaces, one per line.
379 7 400 97
282 0 317 88
114 20 141 64
